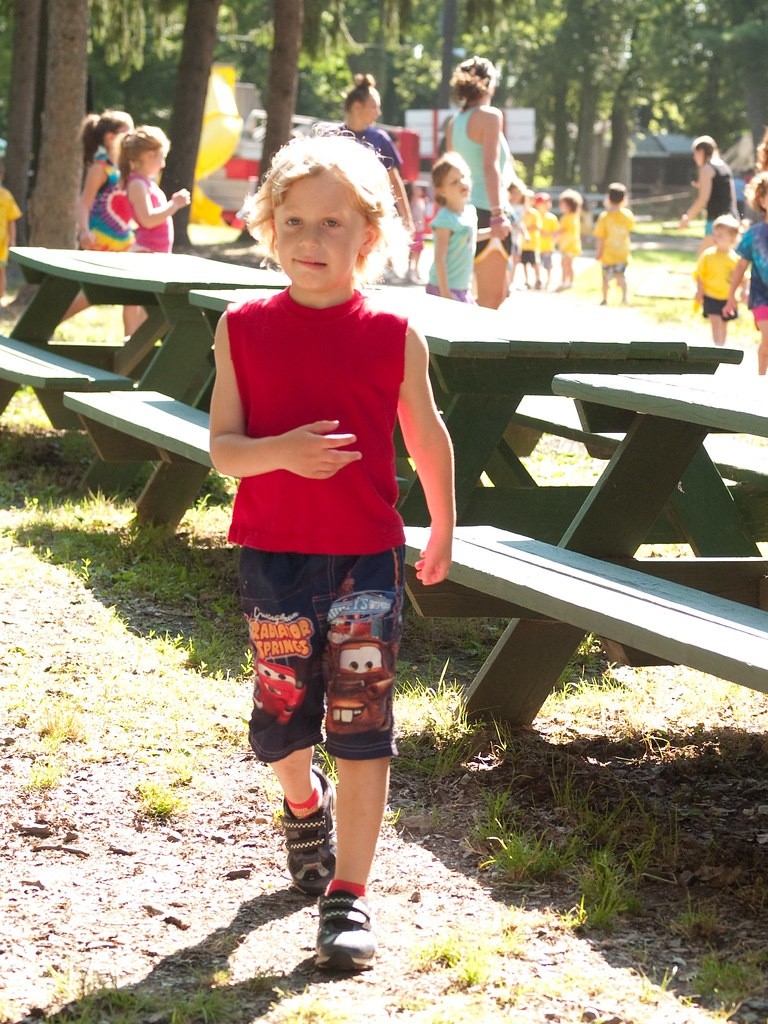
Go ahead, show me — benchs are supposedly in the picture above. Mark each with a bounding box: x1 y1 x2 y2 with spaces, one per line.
1 338 768 696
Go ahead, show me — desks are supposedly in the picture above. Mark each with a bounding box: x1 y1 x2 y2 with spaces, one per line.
411 374 768 730
0 245 291 495
129 287 742 574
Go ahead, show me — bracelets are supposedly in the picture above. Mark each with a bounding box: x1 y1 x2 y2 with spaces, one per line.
682 213 688 221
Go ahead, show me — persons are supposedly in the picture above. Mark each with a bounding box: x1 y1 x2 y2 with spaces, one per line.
206 132 456 973
121 127 192 339
424 56 517 309
593 183 637 306
334 74 427 288
0 163 23 299
509 181 583 291
44 110 135 321
678 133 768 373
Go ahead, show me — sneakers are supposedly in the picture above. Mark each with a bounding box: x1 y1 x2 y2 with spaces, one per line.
281 765 338 893
314 890 377 967
